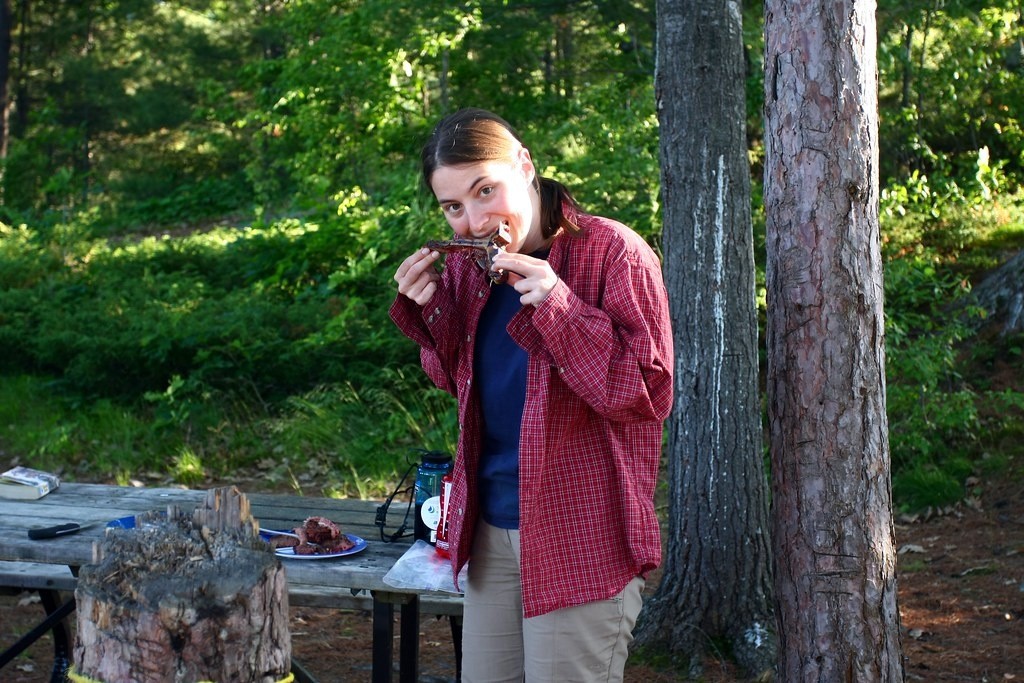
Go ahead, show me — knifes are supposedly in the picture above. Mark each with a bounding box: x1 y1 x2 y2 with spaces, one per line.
27 518 99 540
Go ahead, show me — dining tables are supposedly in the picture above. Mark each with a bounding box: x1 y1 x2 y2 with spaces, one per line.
0 482 466 683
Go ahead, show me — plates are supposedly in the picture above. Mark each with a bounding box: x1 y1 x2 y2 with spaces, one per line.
106 511 192 534
259 529 367 559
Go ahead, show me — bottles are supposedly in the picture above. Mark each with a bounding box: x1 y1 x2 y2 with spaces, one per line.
406 448 454 549
435 464 453 559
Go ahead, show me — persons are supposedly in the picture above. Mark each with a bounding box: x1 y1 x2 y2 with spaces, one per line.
387 107 674 683
